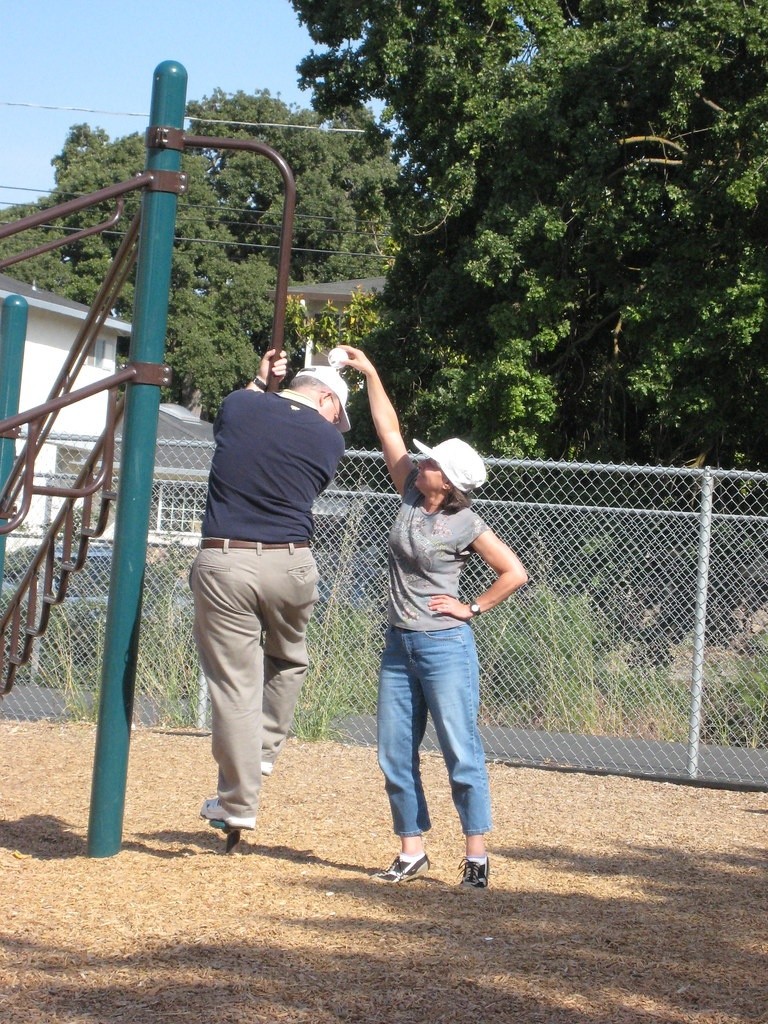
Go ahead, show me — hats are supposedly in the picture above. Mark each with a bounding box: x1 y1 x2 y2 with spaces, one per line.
295 366 350 433
413 438 487 494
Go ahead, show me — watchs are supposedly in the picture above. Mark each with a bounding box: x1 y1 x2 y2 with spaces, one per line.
470 601 482 616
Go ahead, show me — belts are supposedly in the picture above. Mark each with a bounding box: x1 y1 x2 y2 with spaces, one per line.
201 540 310 549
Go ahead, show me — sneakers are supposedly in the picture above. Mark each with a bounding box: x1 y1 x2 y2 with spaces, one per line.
201 798 256 830
261 761 272 776
457 856 490 888
377 853 430 883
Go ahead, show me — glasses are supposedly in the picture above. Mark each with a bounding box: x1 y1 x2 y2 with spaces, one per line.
321 390 341 425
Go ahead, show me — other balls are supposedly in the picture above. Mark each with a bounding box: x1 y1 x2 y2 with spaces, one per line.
325 346 349 371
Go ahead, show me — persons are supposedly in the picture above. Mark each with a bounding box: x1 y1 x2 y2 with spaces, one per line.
190 350 351 830
337 345 527 889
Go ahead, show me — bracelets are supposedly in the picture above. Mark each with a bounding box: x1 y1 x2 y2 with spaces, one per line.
252 377 267 391
